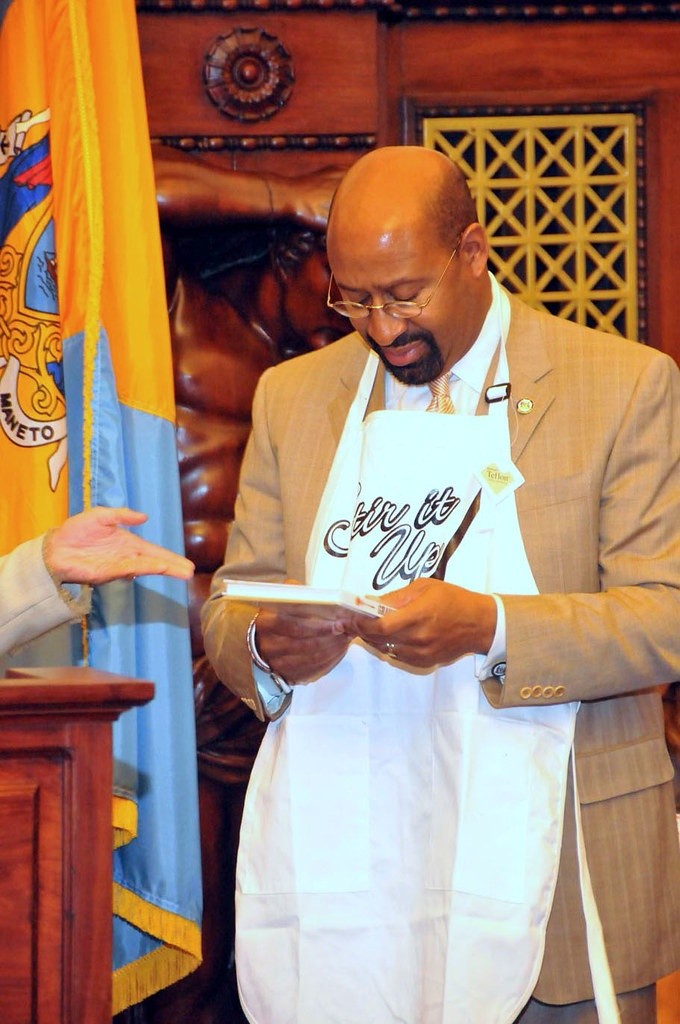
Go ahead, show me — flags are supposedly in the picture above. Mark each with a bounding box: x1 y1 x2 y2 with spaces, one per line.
0 1 206 1023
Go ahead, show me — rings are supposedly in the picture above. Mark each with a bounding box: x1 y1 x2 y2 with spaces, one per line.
386 641 395 651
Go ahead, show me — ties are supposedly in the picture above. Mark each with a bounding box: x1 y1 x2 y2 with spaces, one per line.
423 370 457 415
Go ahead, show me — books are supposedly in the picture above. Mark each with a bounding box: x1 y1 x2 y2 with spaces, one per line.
221 577 396 620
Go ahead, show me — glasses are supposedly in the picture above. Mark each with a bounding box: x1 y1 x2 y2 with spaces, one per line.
326 241 461 320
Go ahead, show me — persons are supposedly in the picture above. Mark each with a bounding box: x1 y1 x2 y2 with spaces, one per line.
198 143 679 1024
0 505 196 659
